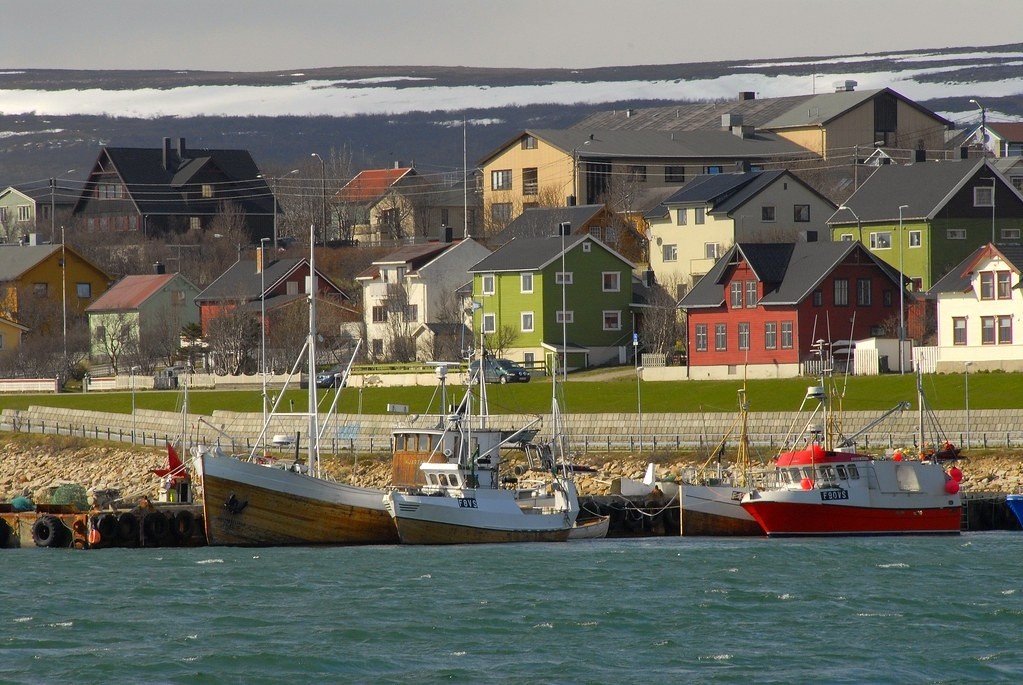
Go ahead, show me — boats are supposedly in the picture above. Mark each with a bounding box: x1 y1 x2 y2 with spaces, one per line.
677 327 769 538
380 292 581 544
567 515 611 540
188 224 400 549
738 309 966 538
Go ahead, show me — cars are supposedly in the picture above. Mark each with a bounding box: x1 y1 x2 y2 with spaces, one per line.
468 357 531 385
672 350 686 364
301 371 347 388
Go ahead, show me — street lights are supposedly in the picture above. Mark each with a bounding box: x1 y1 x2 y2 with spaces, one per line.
259 237 271 458
472 302 485 430
970 99 986 155
214 234 250 261
815 339 827 452
854 141 885 191
965 362 975 451
257 169 300 248
636 366 645 453
51 169 75 244
839 205 862 243
561 221 573 384
311 152 326 248
898 204 909 375
